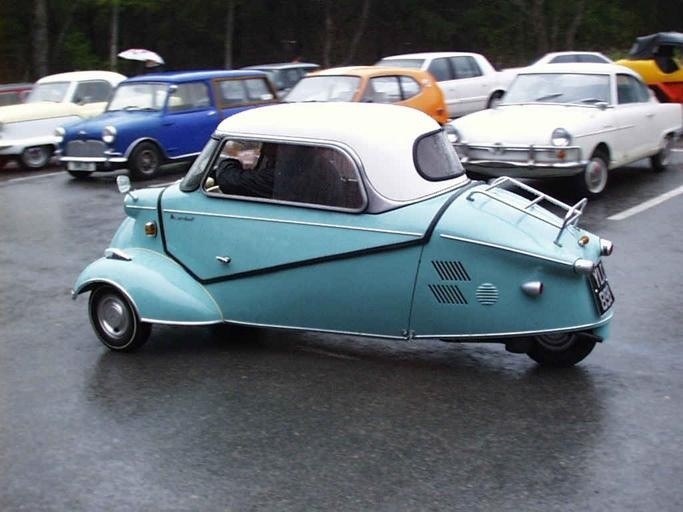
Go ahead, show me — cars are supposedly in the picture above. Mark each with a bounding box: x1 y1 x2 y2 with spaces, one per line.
498 50 617 84
54 68 280 184
0 68 183 171
279 63 449 127
613 32 683 104
220 61 321 103
69 100 615 373
439 62 683 198
371 50 512 119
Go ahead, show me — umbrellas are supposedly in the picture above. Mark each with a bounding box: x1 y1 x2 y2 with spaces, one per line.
114 49 166 66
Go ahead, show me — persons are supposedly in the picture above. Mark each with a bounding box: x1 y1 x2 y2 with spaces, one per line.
215 144 345 207
138 62 161 73
656 42 679 74
